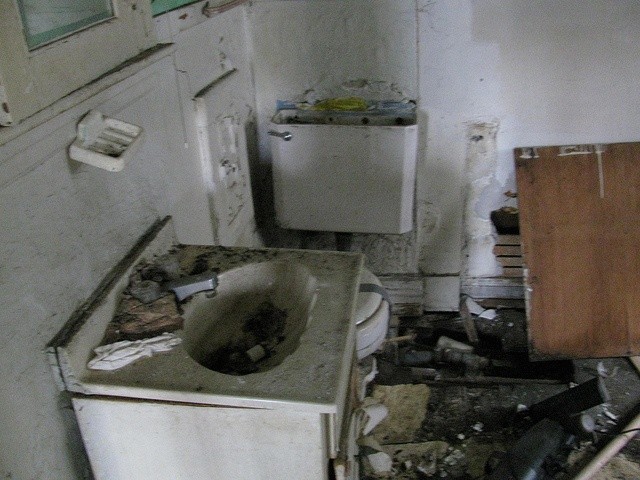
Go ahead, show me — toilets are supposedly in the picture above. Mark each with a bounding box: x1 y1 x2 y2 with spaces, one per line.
268 104 422 383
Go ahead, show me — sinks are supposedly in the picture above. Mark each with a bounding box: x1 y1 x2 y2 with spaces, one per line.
179 260 320 375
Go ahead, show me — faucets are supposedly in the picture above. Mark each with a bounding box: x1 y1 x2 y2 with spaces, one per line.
159 269 218 304
